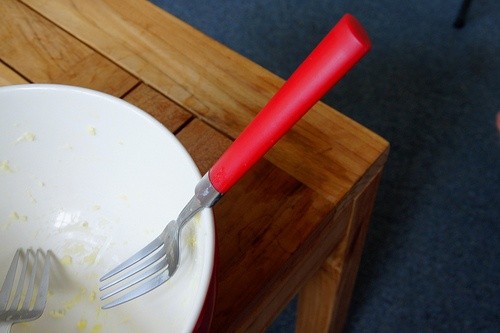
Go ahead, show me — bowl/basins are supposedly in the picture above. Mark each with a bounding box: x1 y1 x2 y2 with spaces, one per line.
0 80 219 333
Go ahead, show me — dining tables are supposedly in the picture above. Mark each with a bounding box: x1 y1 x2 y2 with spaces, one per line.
0 0 392 333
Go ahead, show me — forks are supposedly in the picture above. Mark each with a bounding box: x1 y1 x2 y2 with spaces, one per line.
96 13 369 312
0 245 57 333
